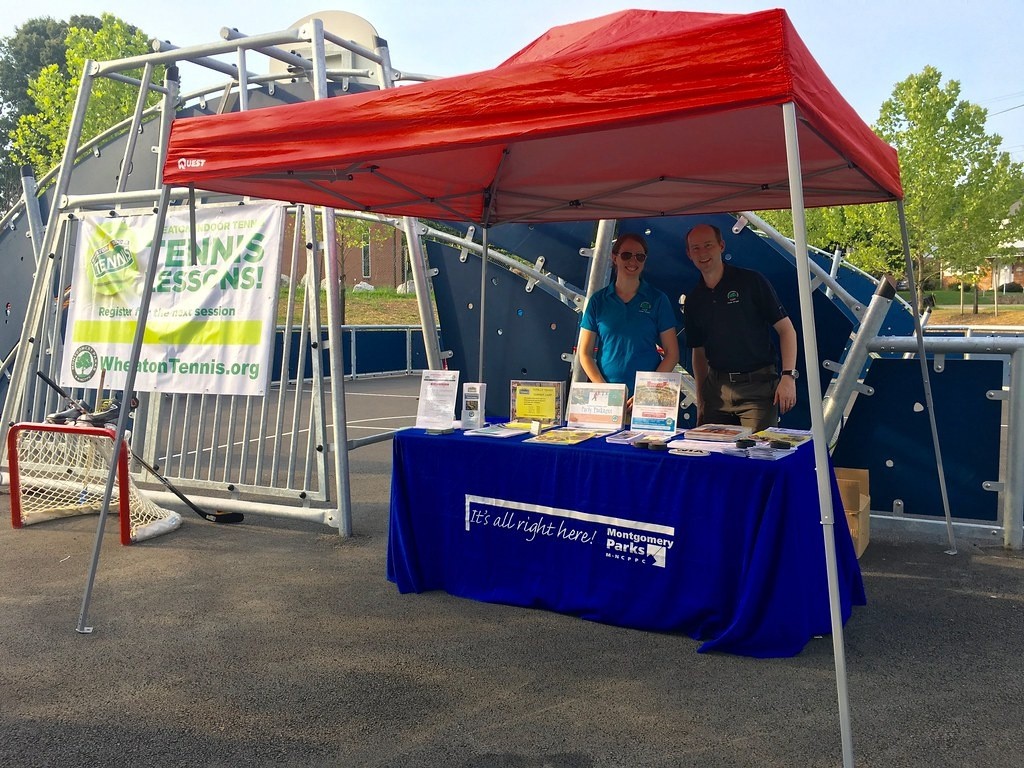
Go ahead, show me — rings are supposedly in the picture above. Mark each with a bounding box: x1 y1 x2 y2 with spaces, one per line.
789 404 794 408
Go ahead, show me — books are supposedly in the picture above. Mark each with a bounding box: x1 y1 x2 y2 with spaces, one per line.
463 424 813 460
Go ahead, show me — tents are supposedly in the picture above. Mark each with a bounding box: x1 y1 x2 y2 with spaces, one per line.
75 9 957 768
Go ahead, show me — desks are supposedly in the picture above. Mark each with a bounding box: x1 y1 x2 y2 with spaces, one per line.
386 417 830 643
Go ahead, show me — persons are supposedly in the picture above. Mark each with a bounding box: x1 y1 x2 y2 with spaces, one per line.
683 224 796 434
579 233 680 425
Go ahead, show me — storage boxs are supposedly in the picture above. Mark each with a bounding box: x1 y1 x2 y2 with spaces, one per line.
831 467 872 559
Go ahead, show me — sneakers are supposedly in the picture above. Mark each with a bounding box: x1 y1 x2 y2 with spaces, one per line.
47 400 94 424
76 400 130 427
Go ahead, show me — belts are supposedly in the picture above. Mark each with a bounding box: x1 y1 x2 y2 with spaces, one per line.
709 363 780 383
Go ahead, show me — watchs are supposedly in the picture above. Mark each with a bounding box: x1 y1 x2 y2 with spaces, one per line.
782 369 799 378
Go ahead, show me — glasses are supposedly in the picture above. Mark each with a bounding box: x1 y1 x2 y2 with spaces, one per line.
616 252 647 263
